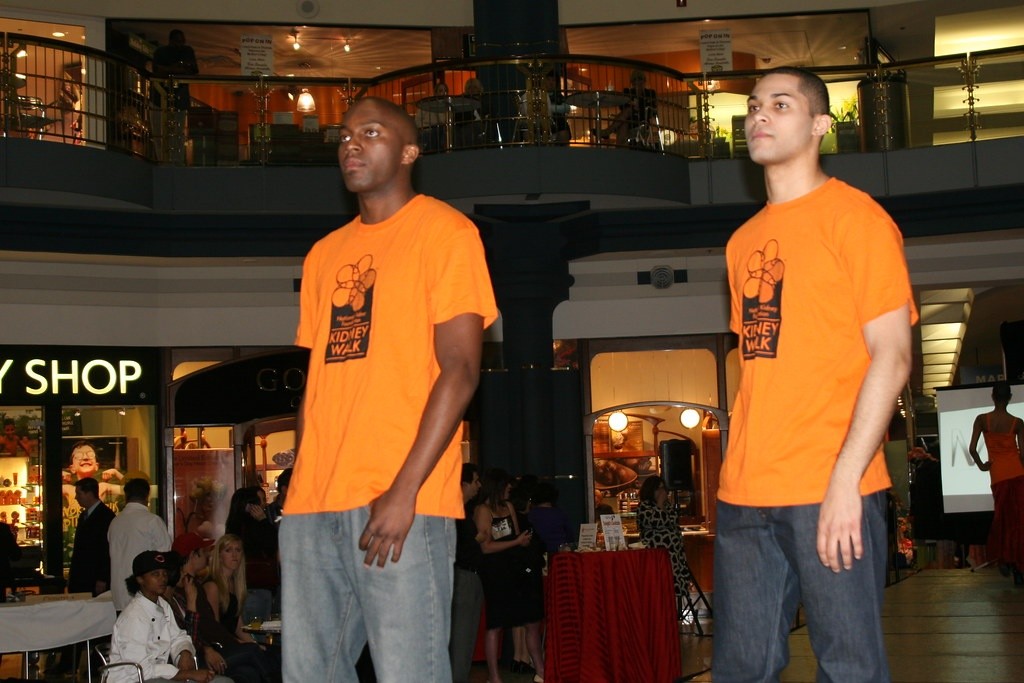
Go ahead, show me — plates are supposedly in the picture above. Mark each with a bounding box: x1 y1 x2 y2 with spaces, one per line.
592 458 637 490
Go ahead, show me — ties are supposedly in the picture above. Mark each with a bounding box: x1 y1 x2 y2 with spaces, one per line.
82 507 87 520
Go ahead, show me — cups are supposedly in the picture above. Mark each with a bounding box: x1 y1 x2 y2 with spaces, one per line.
249 615 263 629
609 537 619 551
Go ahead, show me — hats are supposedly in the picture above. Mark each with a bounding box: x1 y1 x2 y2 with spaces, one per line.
132 550 170 575
171 533 216 559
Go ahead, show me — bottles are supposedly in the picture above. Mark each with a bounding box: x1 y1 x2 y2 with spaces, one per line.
12 578 25 601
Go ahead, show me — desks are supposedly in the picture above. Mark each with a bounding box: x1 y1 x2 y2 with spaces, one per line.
565 91 631 150
544 549 680 683
0 596 117 683
417 95 487 154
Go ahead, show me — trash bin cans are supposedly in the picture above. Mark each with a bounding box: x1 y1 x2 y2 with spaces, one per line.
859 76 912 151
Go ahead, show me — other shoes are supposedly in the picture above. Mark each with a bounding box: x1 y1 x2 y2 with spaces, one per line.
533 674 544 683
1014 575 1022 585
518 660 532 673
998 563 1010 577
510 659 520 671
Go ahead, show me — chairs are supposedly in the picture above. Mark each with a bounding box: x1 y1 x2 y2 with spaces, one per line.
609 100 666 155
96 639 199 683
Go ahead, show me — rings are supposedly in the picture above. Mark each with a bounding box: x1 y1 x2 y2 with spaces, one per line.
220 661 223 663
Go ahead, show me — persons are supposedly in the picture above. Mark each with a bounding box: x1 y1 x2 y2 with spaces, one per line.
0 522 21 576
710 66 920 683
164 468 293 683
149 29 198 167
43 477 117 674
637 475 690 597
591 70 658 149
0 419 30 457
278 97 500 683
970 381 1024 588
61 443 124 526
414 78 504 151
450 464 575 683
107 550 231 683
523 76 572 146
107 477 172 610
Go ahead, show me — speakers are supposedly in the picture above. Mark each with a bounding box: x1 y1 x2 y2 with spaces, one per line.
658 439 694 490
